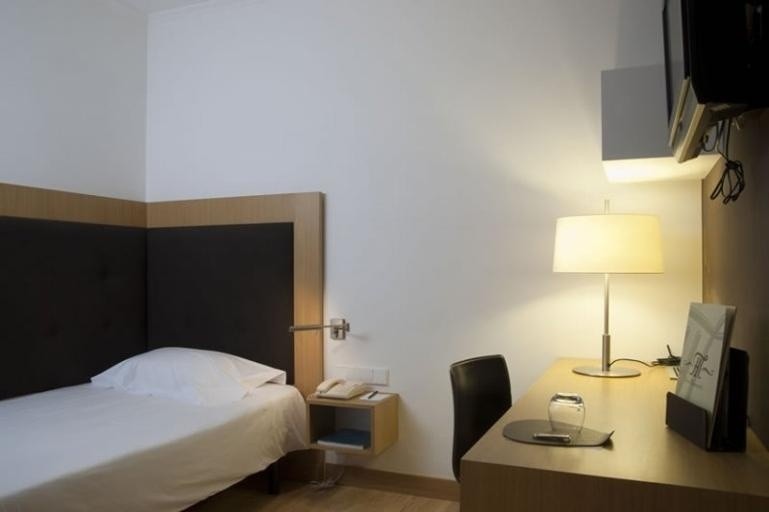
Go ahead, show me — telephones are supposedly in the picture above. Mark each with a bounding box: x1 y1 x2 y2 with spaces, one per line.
315 375 368 399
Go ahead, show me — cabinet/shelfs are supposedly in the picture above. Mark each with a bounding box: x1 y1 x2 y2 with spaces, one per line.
305 390 399 457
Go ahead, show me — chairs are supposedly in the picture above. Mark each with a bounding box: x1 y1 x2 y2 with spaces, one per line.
449 355 513 483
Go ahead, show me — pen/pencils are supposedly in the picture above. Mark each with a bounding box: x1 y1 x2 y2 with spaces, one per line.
368 391 379 400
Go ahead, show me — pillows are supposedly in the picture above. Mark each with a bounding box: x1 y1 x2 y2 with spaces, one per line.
89 346 287 408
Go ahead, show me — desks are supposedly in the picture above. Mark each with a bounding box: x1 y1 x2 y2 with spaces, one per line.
460 354 769 512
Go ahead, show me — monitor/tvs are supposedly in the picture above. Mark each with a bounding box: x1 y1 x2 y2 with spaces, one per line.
660 0 769 163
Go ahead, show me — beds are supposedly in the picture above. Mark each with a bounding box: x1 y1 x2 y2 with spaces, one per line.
0 382 308 512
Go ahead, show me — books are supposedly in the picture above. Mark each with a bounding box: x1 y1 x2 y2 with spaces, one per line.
677 303 738 451
316 426 372 451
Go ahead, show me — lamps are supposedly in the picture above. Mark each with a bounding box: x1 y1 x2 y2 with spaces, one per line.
553 213 664 378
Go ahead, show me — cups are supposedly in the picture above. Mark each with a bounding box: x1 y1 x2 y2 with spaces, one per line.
547 391 586 440
